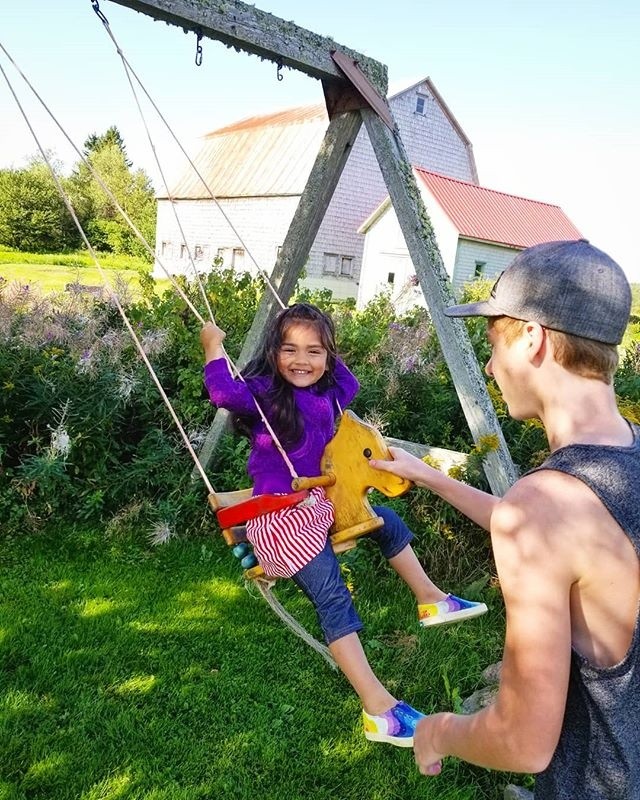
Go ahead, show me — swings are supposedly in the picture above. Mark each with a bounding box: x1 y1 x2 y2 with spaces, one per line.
1 1 411 582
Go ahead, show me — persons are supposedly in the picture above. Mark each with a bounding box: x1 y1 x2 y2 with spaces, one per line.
368 238 640 800
201 305 489 749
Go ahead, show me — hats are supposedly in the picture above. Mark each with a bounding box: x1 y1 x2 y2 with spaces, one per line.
441 237 633 347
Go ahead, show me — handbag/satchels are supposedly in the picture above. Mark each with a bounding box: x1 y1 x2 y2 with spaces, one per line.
243 485 336 581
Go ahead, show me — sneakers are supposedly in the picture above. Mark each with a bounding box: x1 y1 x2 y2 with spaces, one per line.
417 594 488 629
362 701 427 748
